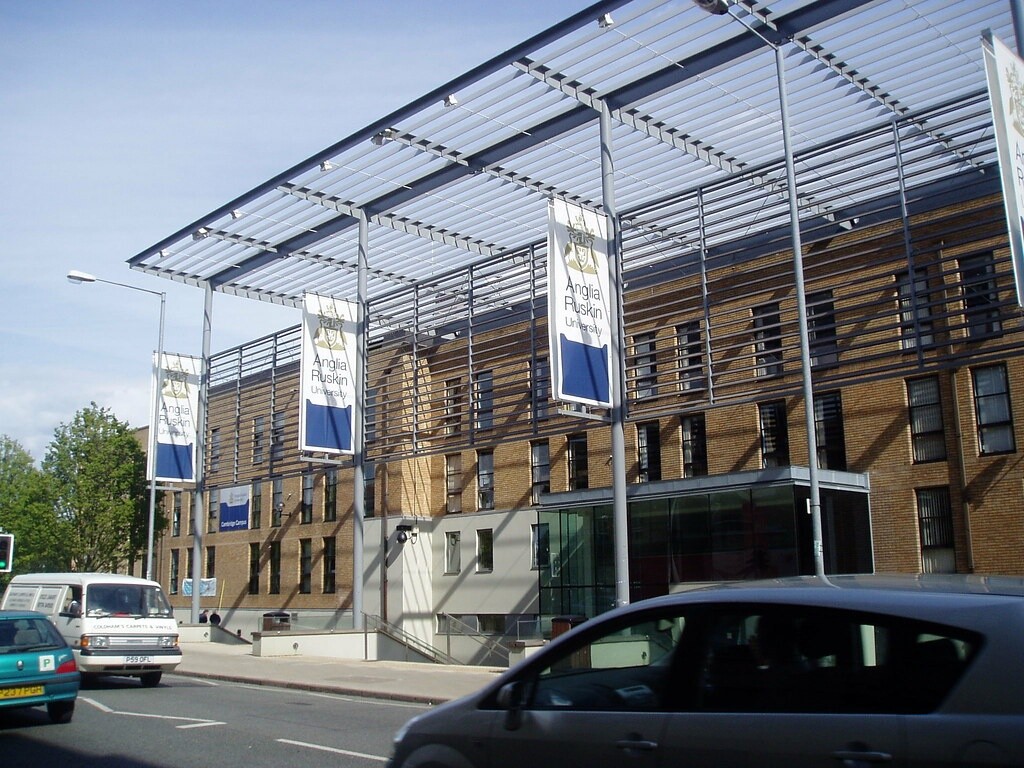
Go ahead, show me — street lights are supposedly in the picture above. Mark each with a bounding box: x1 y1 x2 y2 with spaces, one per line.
66 269 166 581
690 0 824 576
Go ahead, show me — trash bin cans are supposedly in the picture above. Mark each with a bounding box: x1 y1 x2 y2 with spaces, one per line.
262 611 292 631
549 613 592 675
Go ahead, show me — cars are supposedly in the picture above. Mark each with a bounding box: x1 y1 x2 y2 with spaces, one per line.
0 609 80 723
384 572 1024 768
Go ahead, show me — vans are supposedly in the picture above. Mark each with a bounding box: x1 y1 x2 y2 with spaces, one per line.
0 572 184 688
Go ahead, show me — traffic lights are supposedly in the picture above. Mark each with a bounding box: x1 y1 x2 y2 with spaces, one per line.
0 534 14 572
